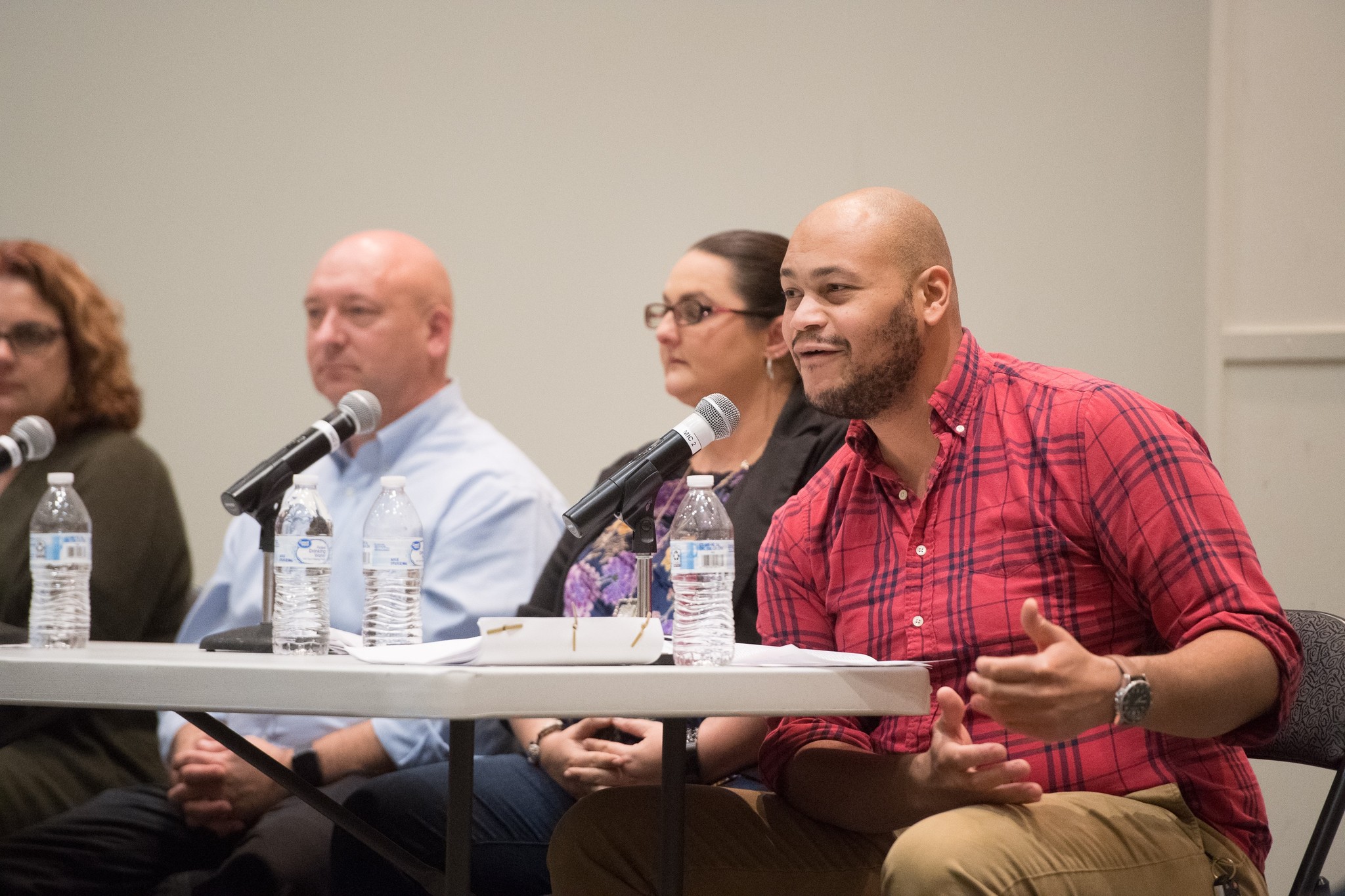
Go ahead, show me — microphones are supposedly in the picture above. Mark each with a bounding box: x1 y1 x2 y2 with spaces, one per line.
221 388 383 516
560 394 740 539
0 415 57 474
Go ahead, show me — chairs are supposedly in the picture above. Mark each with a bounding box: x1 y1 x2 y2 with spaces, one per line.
1222 609 1345 896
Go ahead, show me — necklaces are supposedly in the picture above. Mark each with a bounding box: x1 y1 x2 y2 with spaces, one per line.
611 437 770 619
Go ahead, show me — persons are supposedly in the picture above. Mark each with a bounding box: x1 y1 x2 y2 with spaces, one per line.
323 185 1306 896
0 228 568 895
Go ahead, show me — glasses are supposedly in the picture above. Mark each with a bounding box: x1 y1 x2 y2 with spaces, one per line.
0 321 65 352
645 299 776 329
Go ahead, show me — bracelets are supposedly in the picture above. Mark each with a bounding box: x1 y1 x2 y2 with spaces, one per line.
527 724 567 768
684 727 700 775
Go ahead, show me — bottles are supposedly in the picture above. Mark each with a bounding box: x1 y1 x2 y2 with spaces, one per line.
669 474 735 667
361 475 425 649
26 471 93 651
269 473 334 658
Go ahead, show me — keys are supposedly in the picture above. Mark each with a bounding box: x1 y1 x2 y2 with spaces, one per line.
1210 857 1242 896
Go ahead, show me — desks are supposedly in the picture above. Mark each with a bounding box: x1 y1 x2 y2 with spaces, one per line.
0 641 930 896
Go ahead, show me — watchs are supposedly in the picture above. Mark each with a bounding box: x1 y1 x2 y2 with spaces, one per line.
290 741 326 788
1102 653 1152 728
